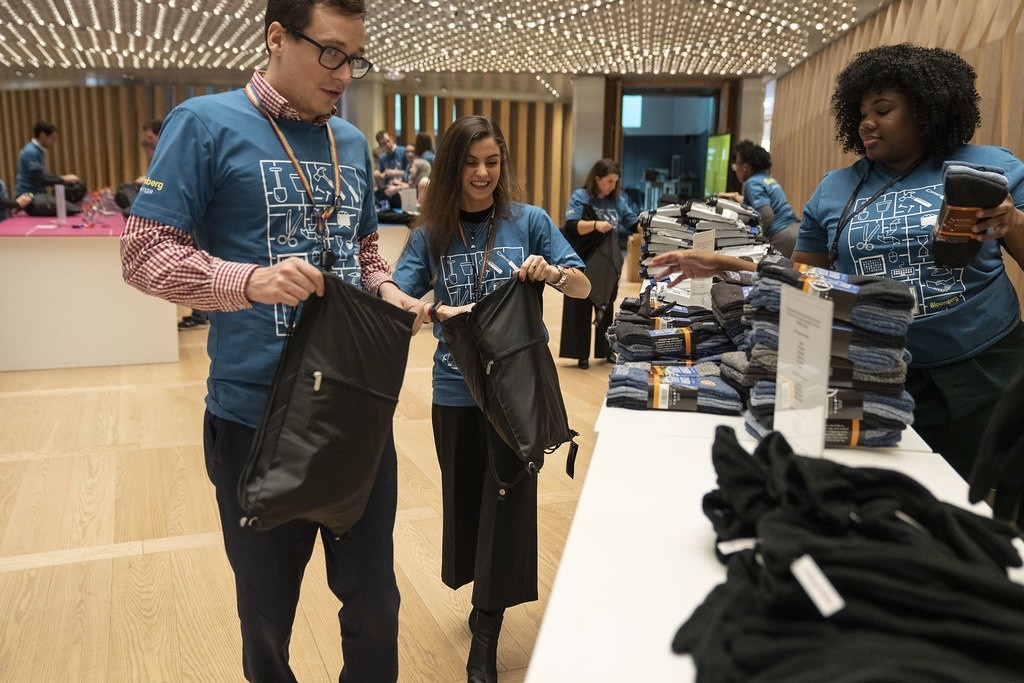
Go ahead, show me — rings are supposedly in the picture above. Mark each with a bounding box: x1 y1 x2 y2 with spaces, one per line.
532 260 537 269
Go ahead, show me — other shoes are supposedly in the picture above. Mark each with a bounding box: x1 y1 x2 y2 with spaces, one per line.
606 353 616 364
578 358 588 369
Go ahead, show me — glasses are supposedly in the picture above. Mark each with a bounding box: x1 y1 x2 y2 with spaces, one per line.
285 26 374 79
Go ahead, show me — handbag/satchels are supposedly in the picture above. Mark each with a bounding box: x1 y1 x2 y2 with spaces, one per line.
117 181 144 216
581 227 624 308
23 193 83 217
51 178 86 201
241 268 416 538
438 270 574 461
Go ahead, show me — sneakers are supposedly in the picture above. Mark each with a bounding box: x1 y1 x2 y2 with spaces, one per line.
178 317 206 330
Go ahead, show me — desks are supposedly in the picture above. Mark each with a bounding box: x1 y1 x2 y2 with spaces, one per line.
0 214 180 371
523 433 1024 683
594 407 934 452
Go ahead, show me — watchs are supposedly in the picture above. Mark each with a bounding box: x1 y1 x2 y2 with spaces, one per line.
548 263 567 288
429 300 448 324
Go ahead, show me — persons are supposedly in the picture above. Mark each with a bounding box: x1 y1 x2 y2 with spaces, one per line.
0 176 33 222
390 111 590 683
140 120 211 329
373 128 436 224
14 118 83 216
558 157 643 369
732 138 802 260
115 0 427 683
647 41 1024 538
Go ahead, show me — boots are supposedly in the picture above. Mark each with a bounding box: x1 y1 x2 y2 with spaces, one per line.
468 604 504 682
